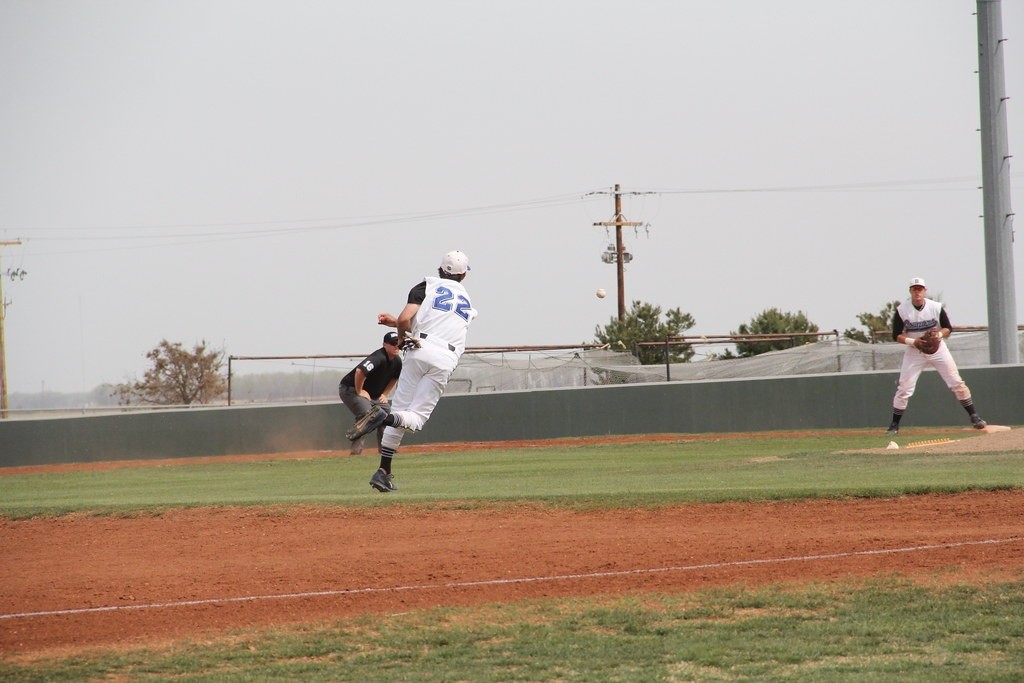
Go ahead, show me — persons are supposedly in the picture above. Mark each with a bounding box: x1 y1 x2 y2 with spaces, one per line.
886 278 986 434
339 331 403 456
369 251 478 493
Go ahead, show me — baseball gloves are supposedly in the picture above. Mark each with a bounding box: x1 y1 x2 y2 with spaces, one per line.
916 327 942 354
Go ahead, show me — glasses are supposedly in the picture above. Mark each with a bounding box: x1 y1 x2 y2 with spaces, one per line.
387 342 397 346
911 287 923 291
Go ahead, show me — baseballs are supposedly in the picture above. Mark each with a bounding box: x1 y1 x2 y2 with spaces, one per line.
597 289 606 298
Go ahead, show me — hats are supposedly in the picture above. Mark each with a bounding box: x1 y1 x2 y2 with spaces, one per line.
440 250 471 275
908 278 925 287
383 332 398 343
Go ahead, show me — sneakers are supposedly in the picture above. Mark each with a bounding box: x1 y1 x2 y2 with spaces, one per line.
369 468 397 492
344 405 388 441
887 421 898 434
970 414 985 429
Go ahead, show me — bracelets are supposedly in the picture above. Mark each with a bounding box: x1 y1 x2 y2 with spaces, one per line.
938 332 942 338
905 338 915 345
